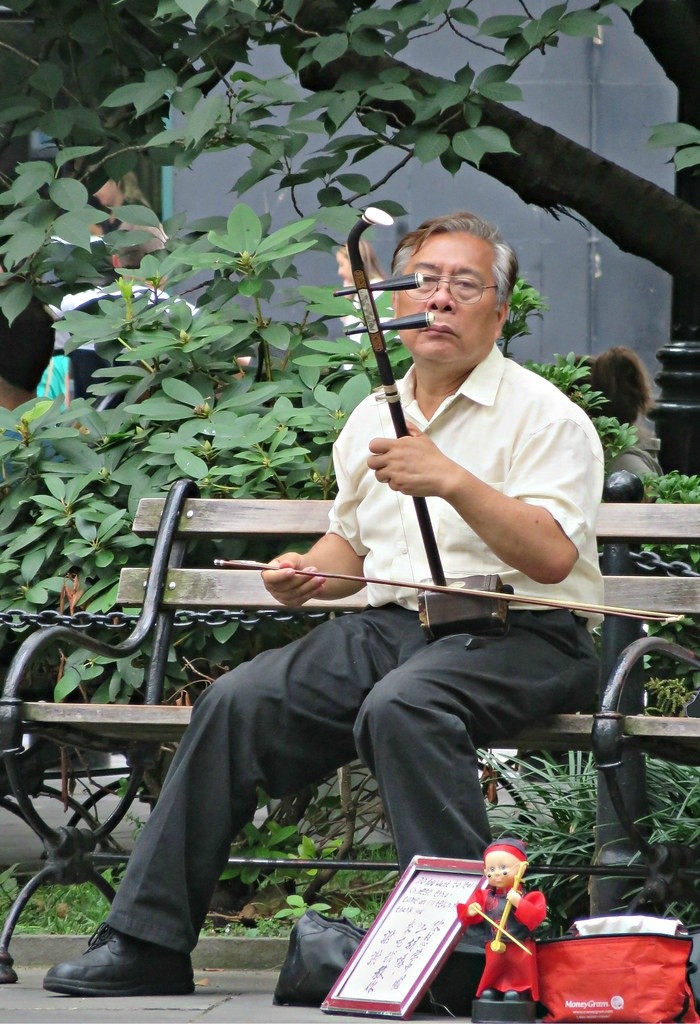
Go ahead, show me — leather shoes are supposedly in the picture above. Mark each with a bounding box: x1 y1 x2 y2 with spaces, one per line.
430 955 485 1017
42 921 196 996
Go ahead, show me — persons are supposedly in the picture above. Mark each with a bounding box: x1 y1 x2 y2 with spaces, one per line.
43 213 607 996
337 239 399 370
457 838 546 1001
0 171 249 480
573 347 663 489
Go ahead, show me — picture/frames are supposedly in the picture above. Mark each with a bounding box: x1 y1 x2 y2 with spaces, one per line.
319 854 495 1021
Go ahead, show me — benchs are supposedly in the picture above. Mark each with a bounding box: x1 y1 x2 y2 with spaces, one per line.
0 477 700 1024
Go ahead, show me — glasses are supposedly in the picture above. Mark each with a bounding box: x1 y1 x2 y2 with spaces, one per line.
404 276 499 305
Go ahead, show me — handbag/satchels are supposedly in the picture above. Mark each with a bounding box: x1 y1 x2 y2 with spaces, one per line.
274 912 430 1014
535 929 700 1024
